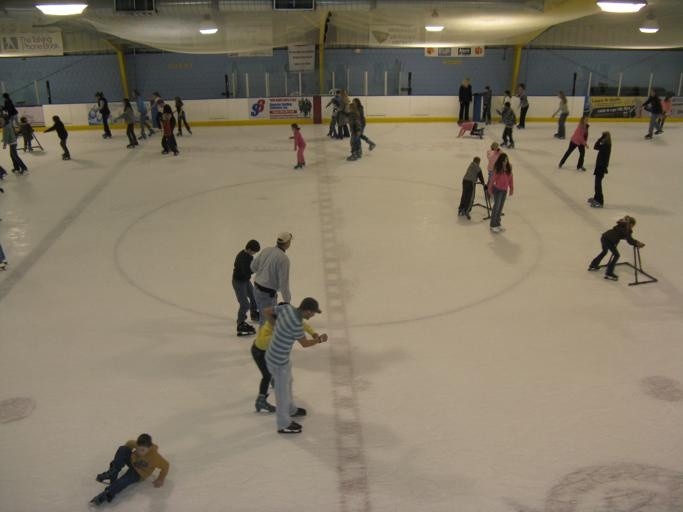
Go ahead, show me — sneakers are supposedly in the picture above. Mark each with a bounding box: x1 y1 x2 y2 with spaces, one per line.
99 471 111 479
290 408 306 416
237 322 255 332
287 421 302 429
91 494 105 504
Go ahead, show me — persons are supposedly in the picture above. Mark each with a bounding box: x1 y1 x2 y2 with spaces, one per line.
587 215 645 280
90 433 170 505
0 88 190 194
289 123 308 169
455 76 529 232
660 93 674 134
249 301 322 412
589 131 612 207
641 89 663 140
323 89 375 161
0 217 10 271
248 231 295 326
263 297 327 434
231 238 261 336
558 113 590 172
552 91 569 140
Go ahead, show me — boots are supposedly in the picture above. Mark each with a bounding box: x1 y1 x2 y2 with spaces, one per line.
256 393 276 412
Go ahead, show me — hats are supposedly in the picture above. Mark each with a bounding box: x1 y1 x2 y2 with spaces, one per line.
277 233 292 244
300 297 322 313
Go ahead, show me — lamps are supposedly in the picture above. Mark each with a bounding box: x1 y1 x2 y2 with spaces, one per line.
32 1 90 18
197 15 220 38
423 4 446 34
595 0 650 17
637 17 661 34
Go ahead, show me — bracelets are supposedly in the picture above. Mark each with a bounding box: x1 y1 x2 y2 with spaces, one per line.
318 336 323 343
